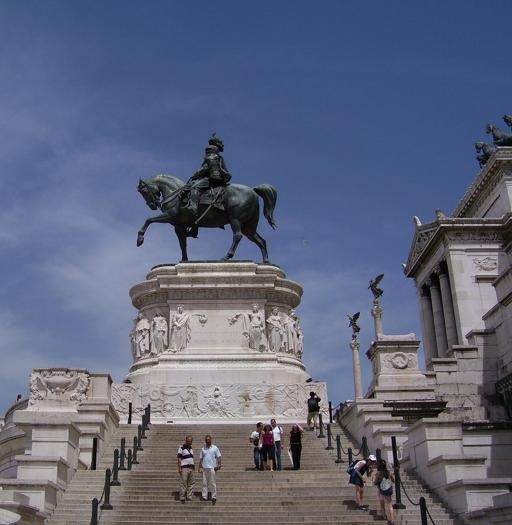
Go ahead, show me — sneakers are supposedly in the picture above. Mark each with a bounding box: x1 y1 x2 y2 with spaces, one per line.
182 497 216 503
356 505 369 511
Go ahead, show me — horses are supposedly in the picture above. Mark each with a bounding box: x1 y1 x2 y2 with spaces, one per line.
136 172 278 264
475 114 512 169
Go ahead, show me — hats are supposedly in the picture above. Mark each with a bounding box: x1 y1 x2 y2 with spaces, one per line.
368 455 378 463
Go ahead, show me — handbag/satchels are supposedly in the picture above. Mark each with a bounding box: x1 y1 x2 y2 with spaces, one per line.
253 437 258 446
288 450 294 466
380 477 393 492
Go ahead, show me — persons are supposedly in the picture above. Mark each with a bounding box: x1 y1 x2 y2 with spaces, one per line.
177 436 196 503
350 455 377 511
307 391 321 429
16 395 22 402
374 459 396 524
368 273 384 298
198 434 221 502
250 419 304 470
348 311 359 335
181 144 224 215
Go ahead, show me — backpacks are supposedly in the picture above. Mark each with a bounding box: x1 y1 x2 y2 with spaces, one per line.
309 398 319 412
347 460 368 474
